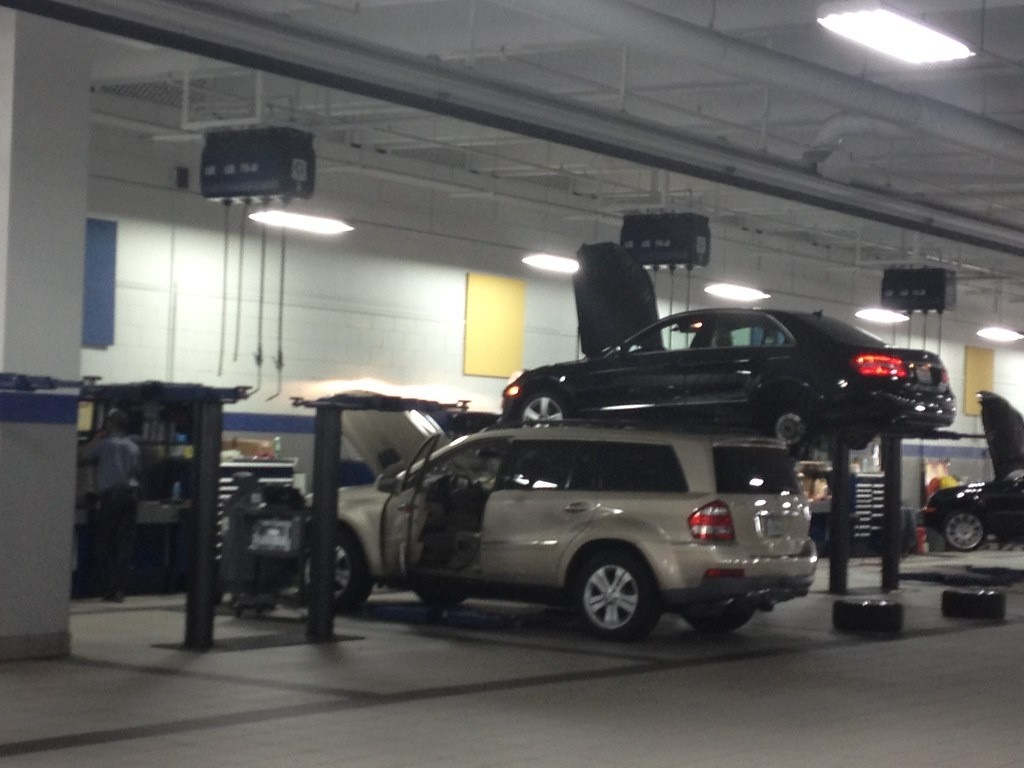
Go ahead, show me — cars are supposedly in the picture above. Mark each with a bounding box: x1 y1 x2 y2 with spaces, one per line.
501 309 958 458
916 391 1023 553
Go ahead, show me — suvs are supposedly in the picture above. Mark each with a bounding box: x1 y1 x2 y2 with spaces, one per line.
287 389 818 645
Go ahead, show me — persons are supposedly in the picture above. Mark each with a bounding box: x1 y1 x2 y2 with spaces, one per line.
76 408 141 604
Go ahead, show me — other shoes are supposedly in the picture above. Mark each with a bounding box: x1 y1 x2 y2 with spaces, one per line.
104 591 123 603
